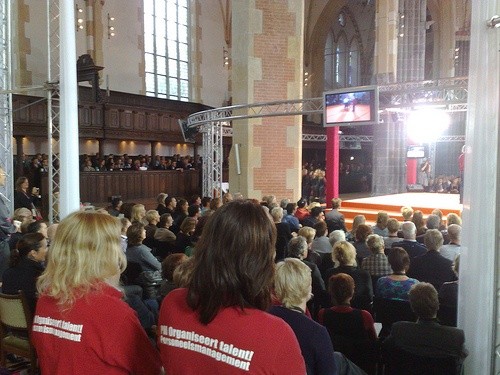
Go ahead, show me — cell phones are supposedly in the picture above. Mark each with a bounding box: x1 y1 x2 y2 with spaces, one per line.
35 188 39 194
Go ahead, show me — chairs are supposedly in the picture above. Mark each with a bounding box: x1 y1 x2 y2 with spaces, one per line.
1 287 41 374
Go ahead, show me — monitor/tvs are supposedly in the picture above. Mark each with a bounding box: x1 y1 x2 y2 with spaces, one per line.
326 89 375 124
406 146 425 158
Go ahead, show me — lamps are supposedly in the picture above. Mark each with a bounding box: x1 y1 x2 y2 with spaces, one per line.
106 13 115 40
76 4 84 33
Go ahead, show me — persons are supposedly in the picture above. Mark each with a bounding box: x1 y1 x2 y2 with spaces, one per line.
436 253 468 327
289 225 324 276
158 252 191 306
412 229 457 291
0 165 21 296
361 234 396 280
156 197 309 375
183 217 215 262
315 273 379 375
169 218 199 258
376 279 467 375
268 256 340 375
377 246 426 325
325 92 371 114
320 229 362 276
281 236 324 313
30 210 163 375
10 231 48 375
123 222 164 290
142 223 163 261
115 282 160 334
324 239 373 315
7 138 474 245
